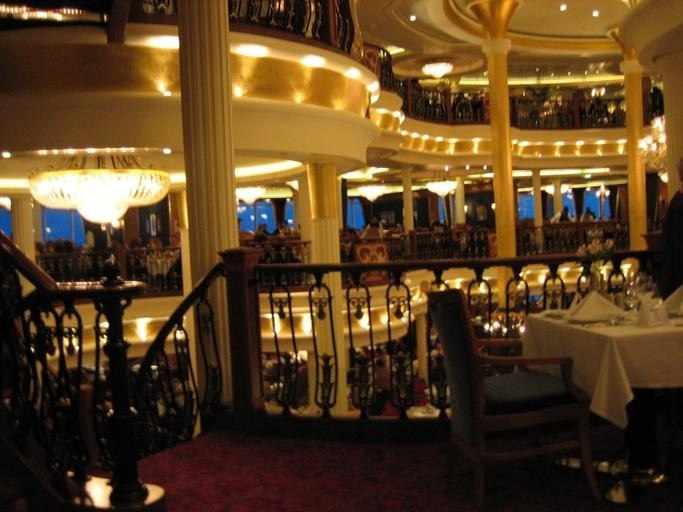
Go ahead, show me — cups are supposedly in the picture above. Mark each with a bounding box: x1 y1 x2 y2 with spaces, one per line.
621 271 656 307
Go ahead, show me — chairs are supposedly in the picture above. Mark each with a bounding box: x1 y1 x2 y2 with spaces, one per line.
428 288 607 512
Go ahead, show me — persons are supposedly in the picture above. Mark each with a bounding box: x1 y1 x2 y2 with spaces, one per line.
359 215 384 243
578 208 595 221
238 220 300 248
35 224 181 294
550 206 575 224
653 157 682 301
